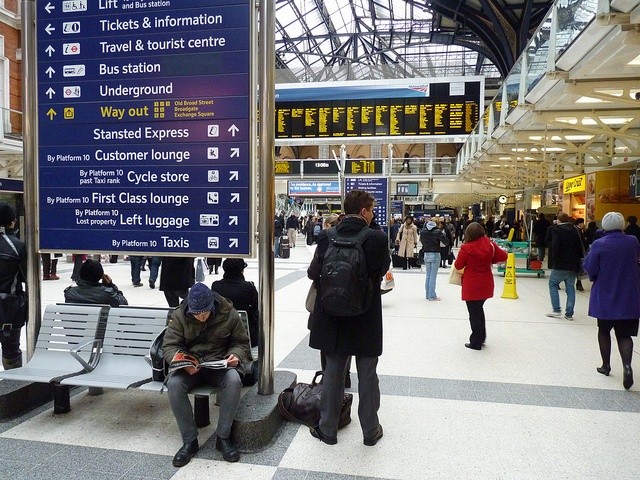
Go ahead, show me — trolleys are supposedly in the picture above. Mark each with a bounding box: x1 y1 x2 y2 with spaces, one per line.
495 238 545 278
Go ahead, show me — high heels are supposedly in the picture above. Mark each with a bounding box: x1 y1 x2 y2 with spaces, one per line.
576 280 583 292
596 365 611 376
623 364 633 389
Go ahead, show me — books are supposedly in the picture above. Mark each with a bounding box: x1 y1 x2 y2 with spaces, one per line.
168 347 228 372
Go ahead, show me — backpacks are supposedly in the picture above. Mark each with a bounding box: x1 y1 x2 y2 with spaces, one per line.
314 226 320 236
320 226 376 316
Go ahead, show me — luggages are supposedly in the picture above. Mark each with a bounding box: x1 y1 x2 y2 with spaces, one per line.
410 247 421 269
392 242 403 267
280 235 290 258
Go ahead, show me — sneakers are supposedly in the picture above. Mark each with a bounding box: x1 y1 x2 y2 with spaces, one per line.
43 274 50 279
134 283 143 287
429 296 441 301
149 279 155 288
50 274 59 280
546 311 562 317
564 314 573 321
443 265 447 268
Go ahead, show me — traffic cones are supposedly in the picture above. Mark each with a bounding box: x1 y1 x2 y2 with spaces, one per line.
501 253 518 299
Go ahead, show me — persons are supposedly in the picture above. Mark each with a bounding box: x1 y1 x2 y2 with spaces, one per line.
396 215 418 270
41 253 51 280
534 213 551 261
306 213 352 389
575 218 590 291
305 215 313 245
399 149 411 173
148 257 163 289
207 257 222 273
70 254 86 282
495 216 511 239
454 221 508 351
301 216 307 235
286 212 301 248
307 187 392 448
419 218 450 302
439 216 453 269
49 253 63 280
582 212 640 390
161 281 251 468
142 256 152 272
624 215 640 236
158 256 194 307
546 212 584 321
587 215 599 244
271 209 286 259
130 256 144 288
63 258 128 307
454 218 462 248
314 210 323 243
299 216 303 232
0 202 27 370
211 257 258 388
493 210 501 223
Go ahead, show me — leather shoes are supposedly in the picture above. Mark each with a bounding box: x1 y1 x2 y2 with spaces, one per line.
310 426 337 444
465 343 482 350
215 435 239 462
173 438 198 467
363 424 383 446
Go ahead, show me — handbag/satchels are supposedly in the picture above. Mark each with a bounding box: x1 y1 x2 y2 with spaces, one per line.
150 326 168 382
278 369 353 430
0 292 27 330
574 257 589 280
448 247 455 265
448 259 467 286
167 260 195 289
394 244 399 251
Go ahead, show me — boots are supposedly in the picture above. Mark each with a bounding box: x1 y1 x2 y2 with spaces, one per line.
408 258 410 269
403 257 407 270
2 353 22 370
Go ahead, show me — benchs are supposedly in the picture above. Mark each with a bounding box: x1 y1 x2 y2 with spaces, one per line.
55 300 267 366
0 303 258 428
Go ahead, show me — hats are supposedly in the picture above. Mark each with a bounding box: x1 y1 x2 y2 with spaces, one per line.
602 211 625 231
574 218 584 225
223 258 247 271
185 283 216 314
79 258 103 281
